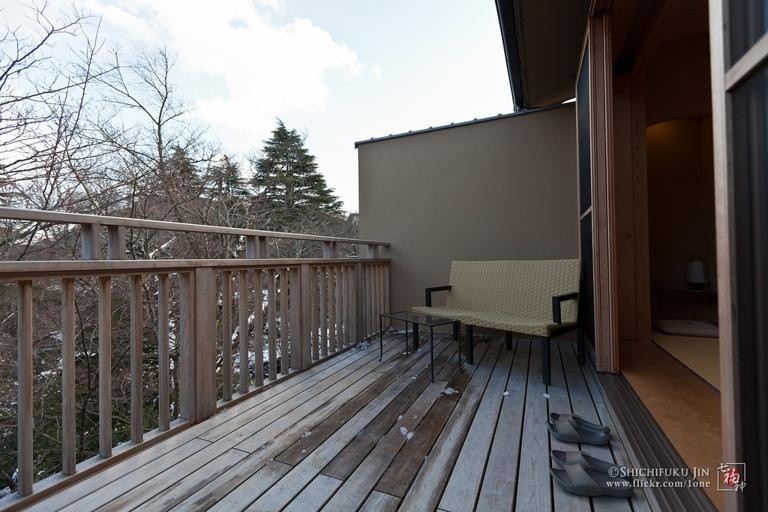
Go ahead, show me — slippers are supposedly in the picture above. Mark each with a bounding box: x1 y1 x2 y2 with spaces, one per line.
546 413 611 446
549 450 634 498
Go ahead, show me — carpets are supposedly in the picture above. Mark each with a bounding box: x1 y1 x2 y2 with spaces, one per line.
650 333 721 392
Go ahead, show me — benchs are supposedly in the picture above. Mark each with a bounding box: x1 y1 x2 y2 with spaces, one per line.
412 259 586 387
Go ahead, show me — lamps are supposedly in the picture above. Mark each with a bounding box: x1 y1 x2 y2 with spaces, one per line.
686 257 711 295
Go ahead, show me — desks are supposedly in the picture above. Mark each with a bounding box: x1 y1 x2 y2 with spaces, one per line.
379 310 464 383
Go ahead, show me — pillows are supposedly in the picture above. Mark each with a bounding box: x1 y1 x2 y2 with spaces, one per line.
652 319 719 338
